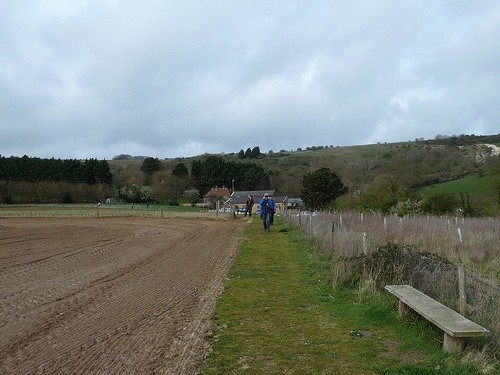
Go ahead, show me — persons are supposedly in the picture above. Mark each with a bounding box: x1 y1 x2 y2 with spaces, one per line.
130 203 134 209
259 193 276 232
146 202 149 209
98 201 101 207
243 195 254 218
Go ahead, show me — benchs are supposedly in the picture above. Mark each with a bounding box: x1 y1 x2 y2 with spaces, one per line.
384 285 491 353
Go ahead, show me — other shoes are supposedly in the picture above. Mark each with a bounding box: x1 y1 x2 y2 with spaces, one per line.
266 229 269 233
264 228 266 231
